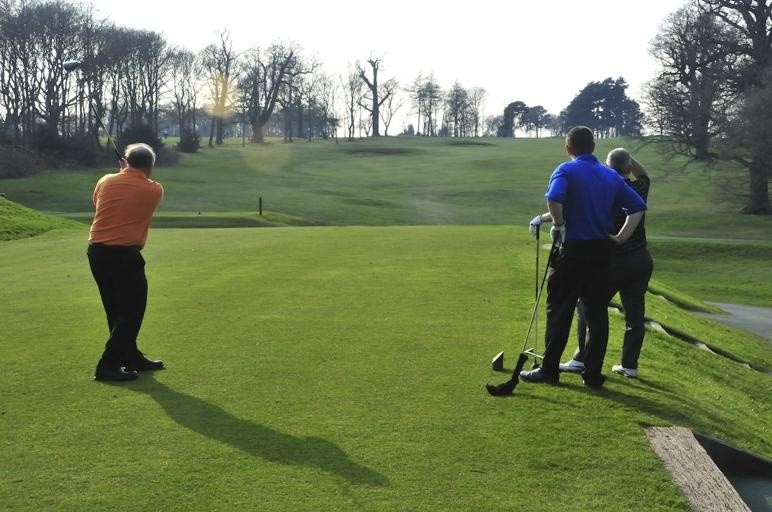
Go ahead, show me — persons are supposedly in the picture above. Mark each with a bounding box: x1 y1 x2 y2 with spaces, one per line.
86 143 165 380
529 147 654 378
520 125 647 390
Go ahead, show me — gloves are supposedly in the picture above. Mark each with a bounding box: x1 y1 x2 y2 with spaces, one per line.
549 222 566 250
528 216 543 237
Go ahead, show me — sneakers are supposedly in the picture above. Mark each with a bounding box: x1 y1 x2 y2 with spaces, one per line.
520 368 544 382
94 369 138 380
558 359 585 371
612 365 636 377
124 358 164 372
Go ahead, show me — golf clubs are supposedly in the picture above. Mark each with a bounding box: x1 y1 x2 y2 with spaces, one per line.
485 231 559 396
532 224 540 368
64 61 123 163
525 348 545 359
